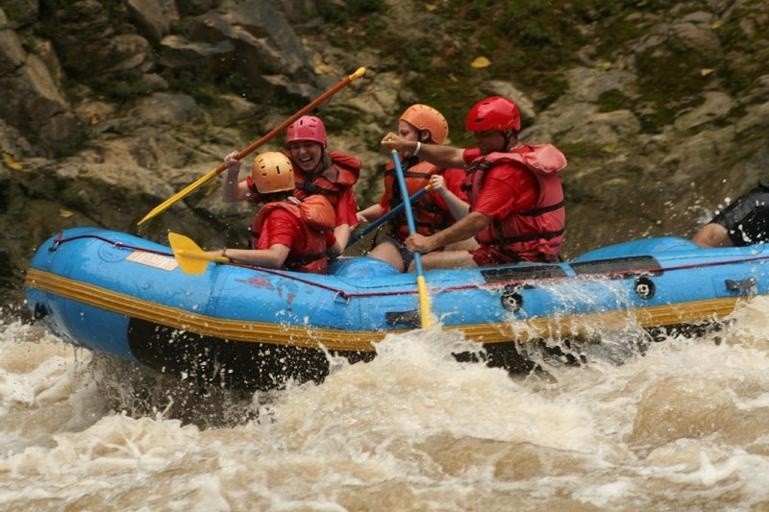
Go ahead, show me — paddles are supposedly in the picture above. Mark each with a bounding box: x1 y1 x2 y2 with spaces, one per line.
166 231 229 274
387 138 433 329
137 66 367 226
344 185 432 250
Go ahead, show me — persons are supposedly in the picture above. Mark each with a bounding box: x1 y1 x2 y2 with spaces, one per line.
378 95 566 274
204 151 334 276
220 113 361 255
357 102 468 274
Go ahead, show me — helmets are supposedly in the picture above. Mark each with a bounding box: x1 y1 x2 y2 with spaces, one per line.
398 103 449 145
464 94 522 152
285 115 328 172
251 151 296 195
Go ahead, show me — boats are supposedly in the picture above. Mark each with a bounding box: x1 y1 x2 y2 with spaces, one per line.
23 226 769 400
